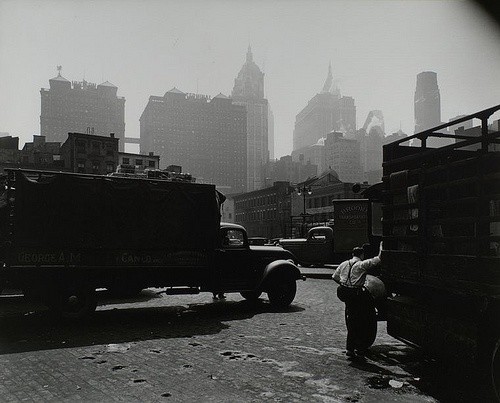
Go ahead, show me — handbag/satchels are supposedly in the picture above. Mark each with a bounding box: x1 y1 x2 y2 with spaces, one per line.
337 283 369 302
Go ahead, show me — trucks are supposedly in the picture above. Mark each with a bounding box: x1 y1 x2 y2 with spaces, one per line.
279 199 383 268
381 103 500 403
1 169 307 320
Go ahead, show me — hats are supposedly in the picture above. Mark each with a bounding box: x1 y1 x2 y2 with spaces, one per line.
353 247 364 253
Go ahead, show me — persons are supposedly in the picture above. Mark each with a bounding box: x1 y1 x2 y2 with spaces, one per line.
332 242 384 357
